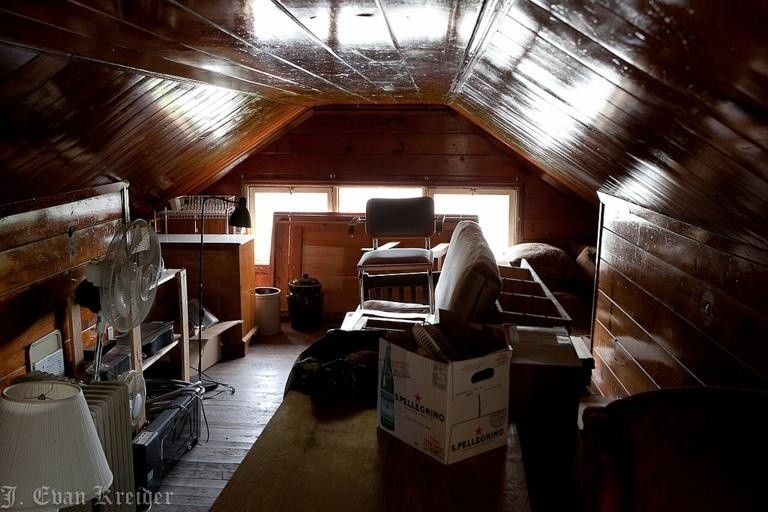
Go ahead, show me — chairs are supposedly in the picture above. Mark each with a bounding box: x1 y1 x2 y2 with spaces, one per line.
356 196 435 314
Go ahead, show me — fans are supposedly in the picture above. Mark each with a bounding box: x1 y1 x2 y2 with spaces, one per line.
82 216 163 381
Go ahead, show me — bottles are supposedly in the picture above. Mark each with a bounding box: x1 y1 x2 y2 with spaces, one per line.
379 345 395 431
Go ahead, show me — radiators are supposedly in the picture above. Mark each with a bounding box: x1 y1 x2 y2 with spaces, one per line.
81 381 139 511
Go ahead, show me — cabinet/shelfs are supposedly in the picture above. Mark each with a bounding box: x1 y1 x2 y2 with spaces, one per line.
64 269 191 422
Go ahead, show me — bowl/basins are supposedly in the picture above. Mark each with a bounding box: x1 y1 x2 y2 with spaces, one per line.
286 272 322 295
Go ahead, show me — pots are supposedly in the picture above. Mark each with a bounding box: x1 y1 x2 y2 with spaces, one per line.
171 297 221 333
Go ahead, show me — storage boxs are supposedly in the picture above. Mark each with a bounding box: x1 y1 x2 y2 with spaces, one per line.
191 316 245 371
377 324 512 465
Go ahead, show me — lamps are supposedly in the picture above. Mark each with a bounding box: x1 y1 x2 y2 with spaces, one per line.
0 381 113 512
190 195 252 394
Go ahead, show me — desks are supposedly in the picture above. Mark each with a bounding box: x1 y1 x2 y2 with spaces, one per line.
153 232 260 357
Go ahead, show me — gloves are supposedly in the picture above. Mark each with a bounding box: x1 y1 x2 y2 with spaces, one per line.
175 194 236 214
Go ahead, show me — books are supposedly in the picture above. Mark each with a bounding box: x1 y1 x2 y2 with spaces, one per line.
168 298 220 339
508 324 558 344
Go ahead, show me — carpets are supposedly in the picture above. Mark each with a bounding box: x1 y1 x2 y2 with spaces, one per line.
208 392 602 511
